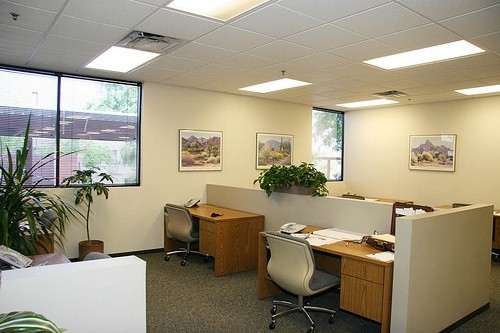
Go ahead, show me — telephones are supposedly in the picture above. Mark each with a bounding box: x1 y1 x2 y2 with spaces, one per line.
185 196 200 208
0 244 34 269
280 222 307 234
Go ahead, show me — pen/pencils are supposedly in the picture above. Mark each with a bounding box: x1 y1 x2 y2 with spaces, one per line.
346 242 349 246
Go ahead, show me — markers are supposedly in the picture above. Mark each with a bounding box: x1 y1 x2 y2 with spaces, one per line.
353 241 362 243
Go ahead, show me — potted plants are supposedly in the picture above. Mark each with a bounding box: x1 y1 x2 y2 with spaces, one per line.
61 166 114 261
253 161 329 198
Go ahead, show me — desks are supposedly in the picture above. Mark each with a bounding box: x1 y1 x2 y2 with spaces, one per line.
18 220 54 256
257 224 394 333
164 204 265 277
434 204 500 249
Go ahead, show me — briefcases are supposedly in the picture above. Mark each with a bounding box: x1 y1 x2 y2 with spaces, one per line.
390 202 434 236
366 234 396 252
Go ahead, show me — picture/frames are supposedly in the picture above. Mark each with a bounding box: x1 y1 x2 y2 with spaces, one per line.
408 134 457 172
255 132 294 170
178 129 223 172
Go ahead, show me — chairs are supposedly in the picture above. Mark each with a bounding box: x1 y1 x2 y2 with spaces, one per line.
165 203 209 266
266 231 341 333
